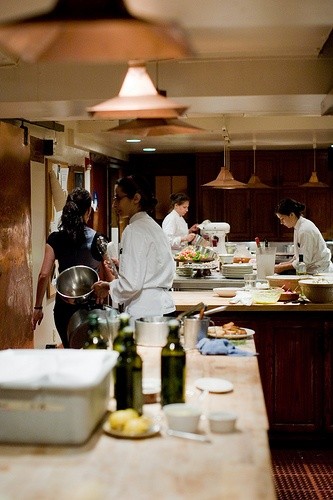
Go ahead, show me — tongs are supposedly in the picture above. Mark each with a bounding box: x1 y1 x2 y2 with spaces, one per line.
174 302 207 320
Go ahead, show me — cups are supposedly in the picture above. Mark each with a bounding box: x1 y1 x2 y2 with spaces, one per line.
243 274 256 290
256 246 276 276
183 319 209 348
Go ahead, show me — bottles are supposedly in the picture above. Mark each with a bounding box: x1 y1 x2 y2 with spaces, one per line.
296 254 306 275
82 313 143 417
160 320 186 406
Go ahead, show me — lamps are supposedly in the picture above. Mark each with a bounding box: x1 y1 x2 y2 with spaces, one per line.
0 0 201 137
300 144 328 188
201 128 273 189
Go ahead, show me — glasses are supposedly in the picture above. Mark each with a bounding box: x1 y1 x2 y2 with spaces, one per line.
113 195 129 205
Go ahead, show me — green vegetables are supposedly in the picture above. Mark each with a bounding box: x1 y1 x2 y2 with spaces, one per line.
181 250 209 260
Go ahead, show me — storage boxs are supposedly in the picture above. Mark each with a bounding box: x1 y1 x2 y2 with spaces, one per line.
0 348 120 446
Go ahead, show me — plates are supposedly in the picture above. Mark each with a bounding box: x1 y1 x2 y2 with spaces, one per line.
222 264 253 278
102 421 159 438
195 377 234 393
208 326 255 339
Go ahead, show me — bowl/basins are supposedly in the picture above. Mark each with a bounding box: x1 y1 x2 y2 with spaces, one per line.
54 266 100 306
134 316 181 348
278 291 300 300
205 409 237 433
219 254 234 263
213 287 244 297
298 280 333 302
251 290 282 304
162 402 202 434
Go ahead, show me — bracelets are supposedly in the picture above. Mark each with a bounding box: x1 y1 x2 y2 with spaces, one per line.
34 306 43 310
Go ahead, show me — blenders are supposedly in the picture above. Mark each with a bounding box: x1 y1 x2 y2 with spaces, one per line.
191 221 230 253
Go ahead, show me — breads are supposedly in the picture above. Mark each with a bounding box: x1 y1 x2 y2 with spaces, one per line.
109 408 151 436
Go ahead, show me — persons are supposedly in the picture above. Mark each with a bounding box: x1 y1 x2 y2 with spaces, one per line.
162 193 200 254
274 197 332 275
33 187 115 349
92 176 176 328
